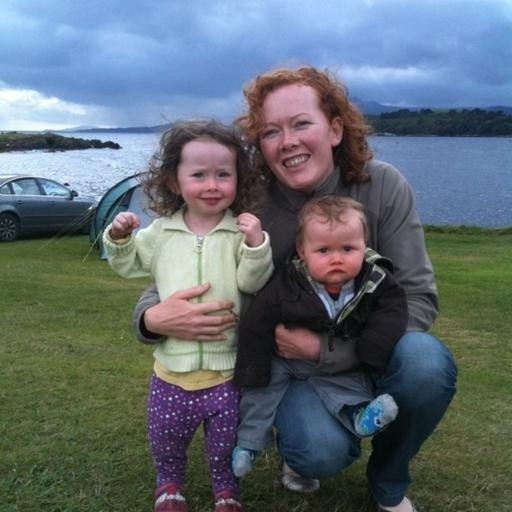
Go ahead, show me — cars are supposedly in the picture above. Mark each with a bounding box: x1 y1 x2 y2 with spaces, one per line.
0 175 95 242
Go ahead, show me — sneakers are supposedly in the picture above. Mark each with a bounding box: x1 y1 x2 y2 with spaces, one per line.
279 457 320 494
210 491 244 512
153 482 190 512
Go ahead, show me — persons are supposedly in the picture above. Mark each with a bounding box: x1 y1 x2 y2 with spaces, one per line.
96 119 276 512
133 66 458 512
232 194 400 482
